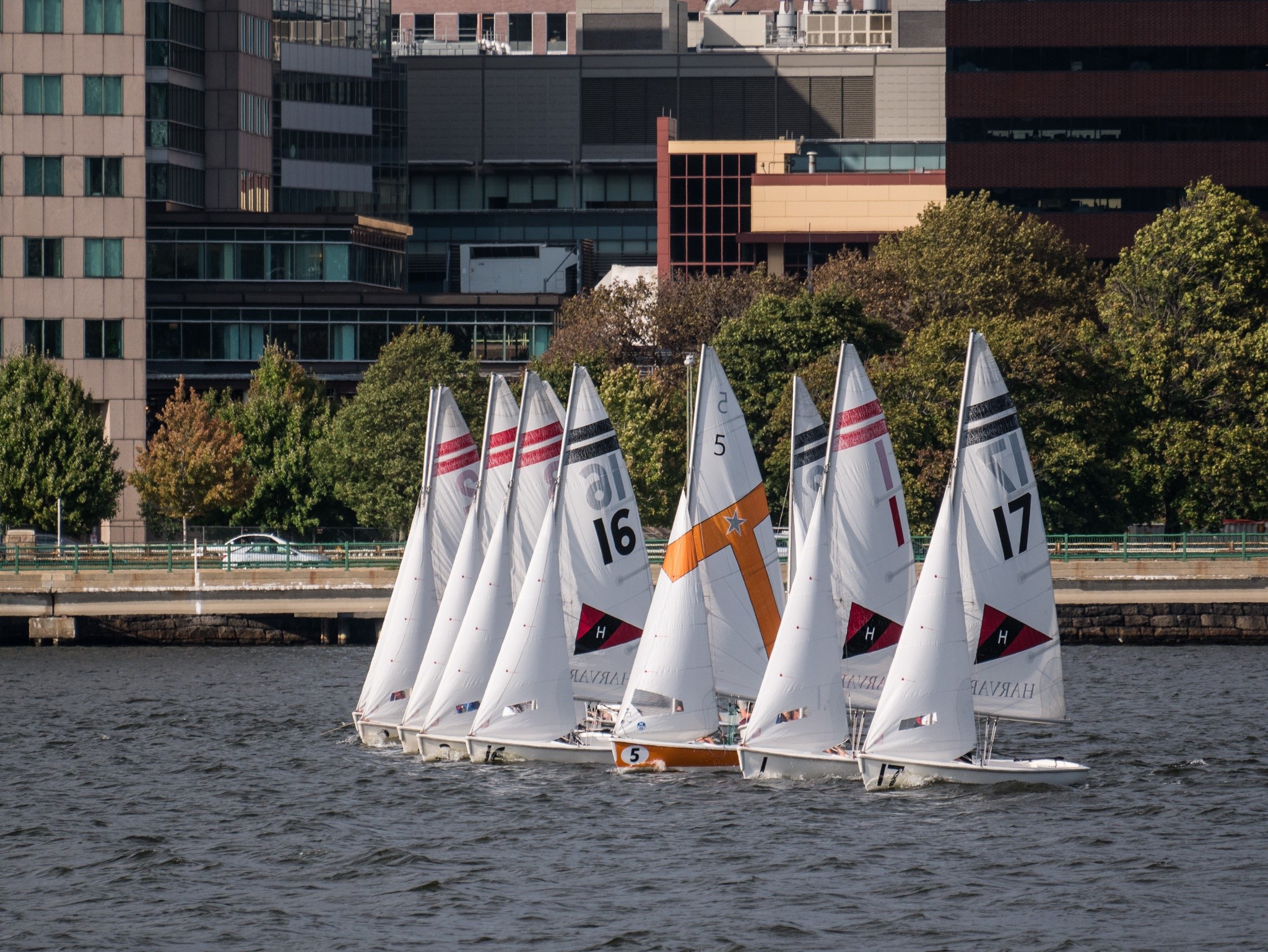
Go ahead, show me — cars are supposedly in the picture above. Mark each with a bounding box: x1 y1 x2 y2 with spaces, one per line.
34 534 86 551
198 533 296 554
220 542 331 567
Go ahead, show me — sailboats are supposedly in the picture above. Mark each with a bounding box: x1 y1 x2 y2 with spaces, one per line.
730 332 923 787
416 366 567 764
600 342 790 781
851 328 1093 791
350 369 520 761
458 361 658 766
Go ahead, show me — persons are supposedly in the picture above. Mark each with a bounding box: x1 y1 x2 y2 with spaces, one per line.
677 697 800 746
